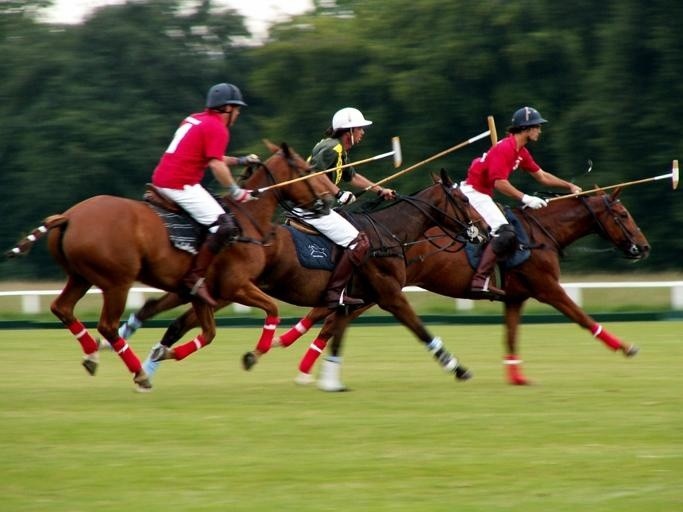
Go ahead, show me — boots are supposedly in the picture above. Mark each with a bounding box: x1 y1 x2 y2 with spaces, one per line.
467 223 517 297
319 231 372 306
178 211 238 307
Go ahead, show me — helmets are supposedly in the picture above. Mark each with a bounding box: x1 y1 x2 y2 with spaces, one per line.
508 105 547 130
332 107 373 132
206 83 249 108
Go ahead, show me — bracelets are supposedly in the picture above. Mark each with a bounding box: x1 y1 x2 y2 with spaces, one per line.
377 185 387 196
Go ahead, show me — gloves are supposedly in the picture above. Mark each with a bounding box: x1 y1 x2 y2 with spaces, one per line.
240 153 260 166
334 189 358 207
520 194 548 210
226 182 257 204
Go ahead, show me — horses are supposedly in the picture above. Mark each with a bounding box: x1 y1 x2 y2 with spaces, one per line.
262 182 653 385
101 168 492 393
0 139 338 390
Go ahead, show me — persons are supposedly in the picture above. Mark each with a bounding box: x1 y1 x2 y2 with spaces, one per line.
457 104 581 300
150 83 258 305
290 107 401 305
